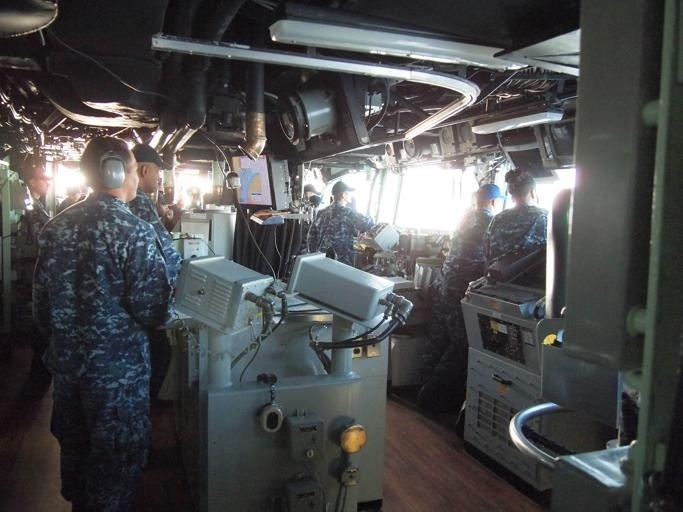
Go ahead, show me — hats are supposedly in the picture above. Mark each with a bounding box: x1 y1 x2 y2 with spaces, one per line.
131 144 171 170
476 184 507 199
304 185 320 195
332 181 354 193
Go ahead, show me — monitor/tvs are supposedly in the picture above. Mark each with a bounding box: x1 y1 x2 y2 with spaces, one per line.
544 119 575 164
503 133 560 182
229 152 276 210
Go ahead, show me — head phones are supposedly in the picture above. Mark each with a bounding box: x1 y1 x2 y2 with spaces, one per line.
99 138 125 189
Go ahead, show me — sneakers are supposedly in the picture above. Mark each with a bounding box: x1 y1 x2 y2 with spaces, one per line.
150 398 174 414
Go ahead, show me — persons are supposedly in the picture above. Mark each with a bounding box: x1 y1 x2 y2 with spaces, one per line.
13 140 182 406
33 137 173 510
299 181 373 267
415 170 548 407
